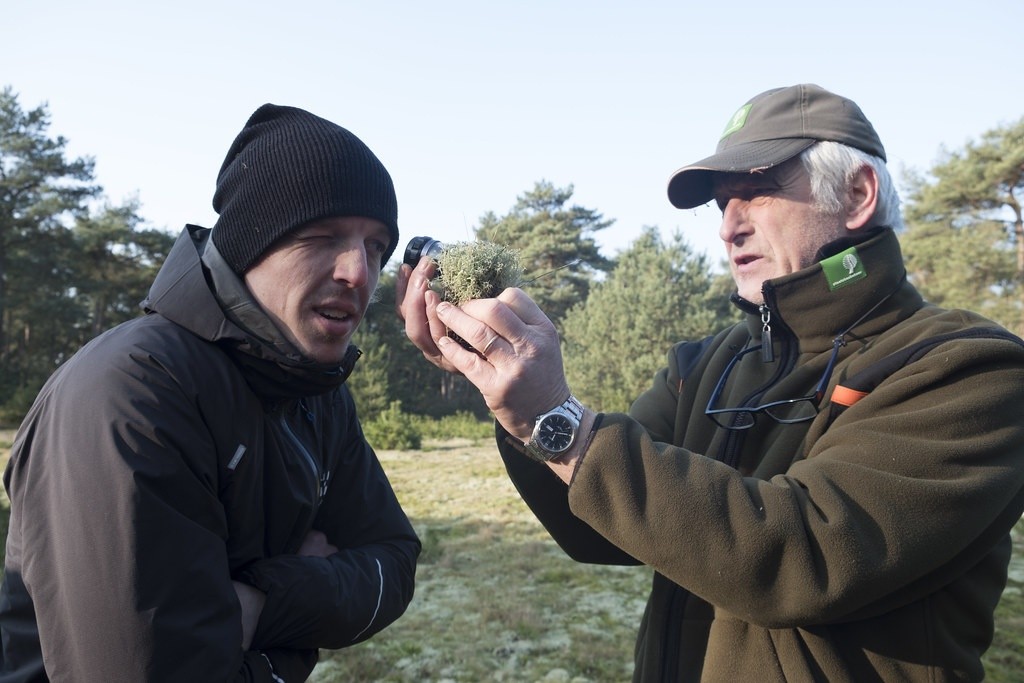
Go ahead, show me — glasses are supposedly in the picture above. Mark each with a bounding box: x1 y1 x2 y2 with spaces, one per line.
706 332 840 431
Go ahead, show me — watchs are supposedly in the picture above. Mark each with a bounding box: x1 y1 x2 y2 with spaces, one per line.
521 395 587 461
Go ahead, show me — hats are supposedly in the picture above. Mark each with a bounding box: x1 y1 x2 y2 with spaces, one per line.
667 81 888 211
211 102 400 284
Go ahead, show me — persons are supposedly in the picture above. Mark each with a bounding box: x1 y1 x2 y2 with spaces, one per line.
0 99 420 683
394 82 1023 683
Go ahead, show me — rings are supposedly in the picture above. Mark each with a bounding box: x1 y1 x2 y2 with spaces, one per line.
482 334 500 355
